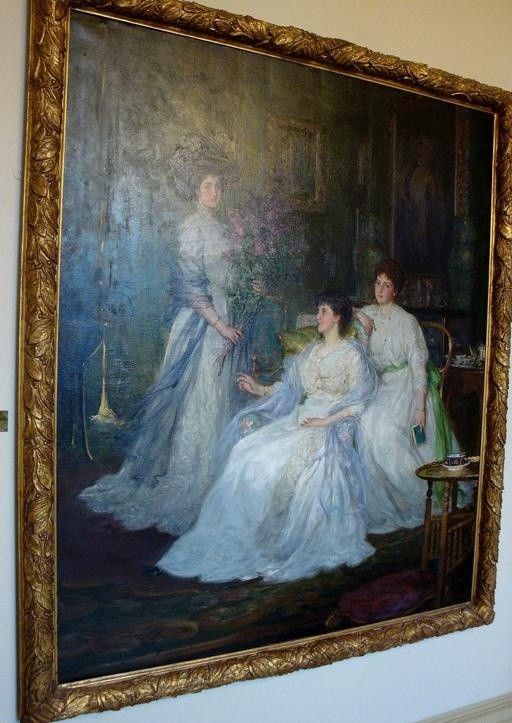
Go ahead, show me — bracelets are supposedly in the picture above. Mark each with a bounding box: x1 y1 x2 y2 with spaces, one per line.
416 407 425 412
212 316 222 329
264 386 267 396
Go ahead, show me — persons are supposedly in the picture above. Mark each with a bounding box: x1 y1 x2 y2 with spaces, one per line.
352 256 475 536
154 290 376 586
76 175 245 539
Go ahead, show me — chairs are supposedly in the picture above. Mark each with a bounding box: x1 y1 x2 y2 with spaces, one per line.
294 310 370 357
57 265 108 463
413 319 453 402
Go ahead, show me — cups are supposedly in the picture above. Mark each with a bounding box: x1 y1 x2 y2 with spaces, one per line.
455 354 474 365
469 345 485 362
446 452 466 464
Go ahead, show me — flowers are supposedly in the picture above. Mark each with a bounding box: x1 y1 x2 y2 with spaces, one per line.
216 190 309 376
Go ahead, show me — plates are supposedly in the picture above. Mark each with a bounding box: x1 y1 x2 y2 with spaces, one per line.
440 460 469 470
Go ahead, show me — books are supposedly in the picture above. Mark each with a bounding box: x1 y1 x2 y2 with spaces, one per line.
411 424 426 445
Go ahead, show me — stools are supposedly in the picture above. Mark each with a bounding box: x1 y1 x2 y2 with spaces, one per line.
324 570 451 632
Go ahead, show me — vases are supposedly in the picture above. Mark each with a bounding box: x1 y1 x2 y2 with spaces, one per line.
346 211 383 303
447 214 478 312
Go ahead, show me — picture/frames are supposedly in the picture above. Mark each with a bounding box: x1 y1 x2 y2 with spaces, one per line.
268 115 329 216
384 97 471 312
14 1 511 721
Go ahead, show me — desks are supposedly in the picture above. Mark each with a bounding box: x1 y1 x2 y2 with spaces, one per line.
441 360 484 440
416 459 480 608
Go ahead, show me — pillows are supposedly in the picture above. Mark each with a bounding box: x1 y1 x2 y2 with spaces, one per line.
276 324 358 361
